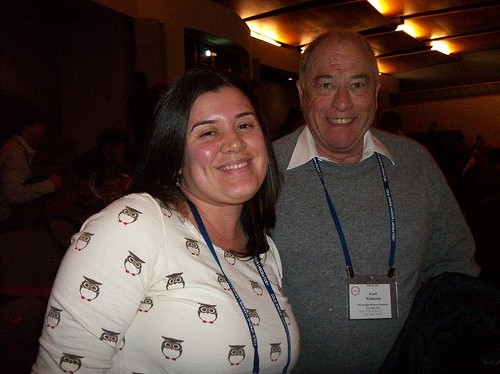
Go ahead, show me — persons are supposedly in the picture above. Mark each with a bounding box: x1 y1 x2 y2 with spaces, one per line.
273 29 481 374
31 68 300 374
0 110 126 228
